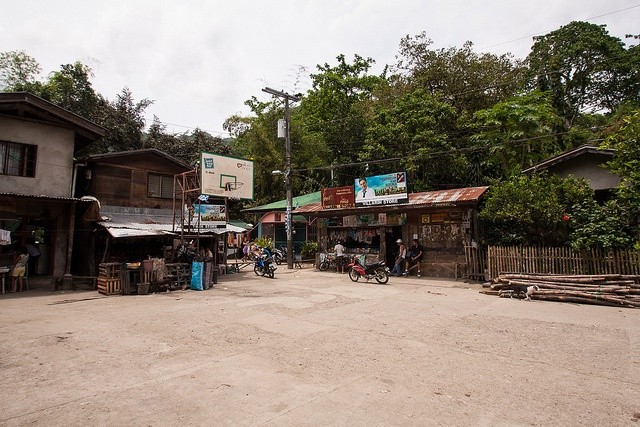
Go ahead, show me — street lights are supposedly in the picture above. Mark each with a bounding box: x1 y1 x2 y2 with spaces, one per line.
272 171 294 270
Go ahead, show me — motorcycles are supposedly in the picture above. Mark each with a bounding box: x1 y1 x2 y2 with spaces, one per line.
255 255 278 279
349 255 391 285
320 250 365 273
263 246 283 266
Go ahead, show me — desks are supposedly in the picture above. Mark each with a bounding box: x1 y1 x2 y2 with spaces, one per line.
0 267 10 295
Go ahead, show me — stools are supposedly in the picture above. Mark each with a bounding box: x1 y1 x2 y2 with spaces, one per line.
63 274 73 294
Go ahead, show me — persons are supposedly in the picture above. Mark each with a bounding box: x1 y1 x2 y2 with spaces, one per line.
245 240 250 260
240 237 250 261
335 240 346 274
8 243 29 293
402 238 423 279
356 178 377 200
390 238 406 277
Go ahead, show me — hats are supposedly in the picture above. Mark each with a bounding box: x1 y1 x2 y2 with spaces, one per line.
396 239 403 243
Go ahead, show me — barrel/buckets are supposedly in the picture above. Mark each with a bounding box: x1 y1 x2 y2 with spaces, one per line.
143 260 154 272
137 283 149 295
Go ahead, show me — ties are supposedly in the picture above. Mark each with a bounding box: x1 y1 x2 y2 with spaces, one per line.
362 191 367 197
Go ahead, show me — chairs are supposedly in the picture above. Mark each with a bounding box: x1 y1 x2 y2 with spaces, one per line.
14 263 30 292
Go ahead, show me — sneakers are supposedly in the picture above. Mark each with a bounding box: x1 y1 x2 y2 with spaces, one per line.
403 270 409 275
417 270 421 278
